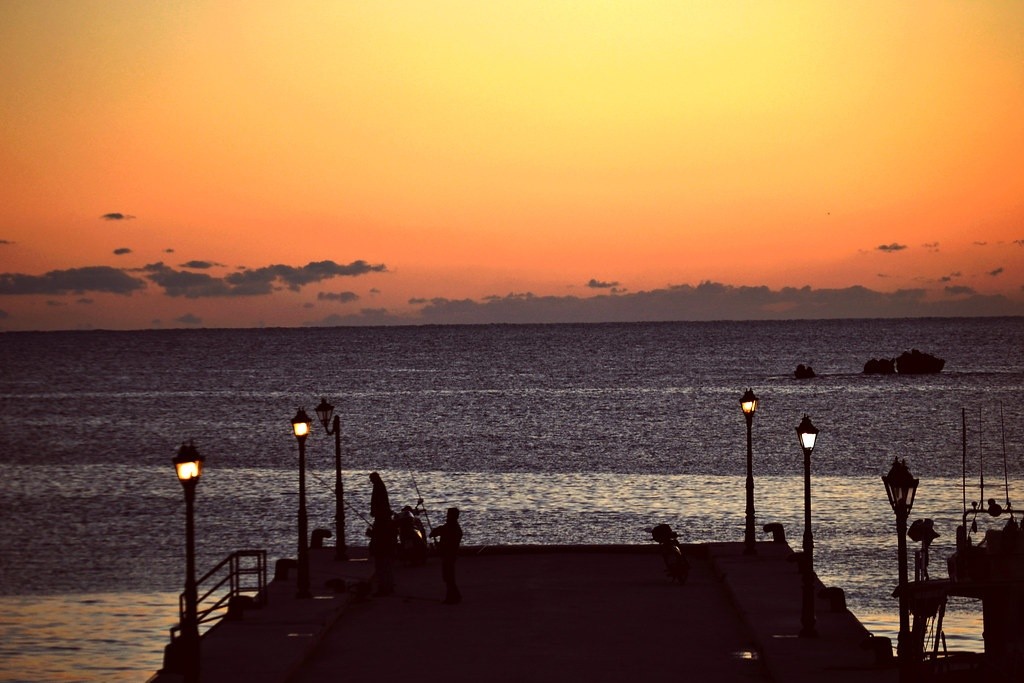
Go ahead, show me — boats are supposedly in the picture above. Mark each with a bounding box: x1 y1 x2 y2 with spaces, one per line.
895 350 947 374
892 397 1024 602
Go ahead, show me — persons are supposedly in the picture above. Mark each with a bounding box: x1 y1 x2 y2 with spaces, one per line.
429 508 462 605
369 472 390 552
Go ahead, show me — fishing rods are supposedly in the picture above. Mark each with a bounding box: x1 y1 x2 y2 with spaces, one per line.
999 399 1012 510
288 452 374 527
977 403 985 510
400 445 438 544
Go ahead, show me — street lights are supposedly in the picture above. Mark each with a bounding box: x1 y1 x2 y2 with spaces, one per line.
173 439 205 676
793 414 822 644
886 458 921 675
313 398 354 565
739 383 761 543
289 407 313 599
